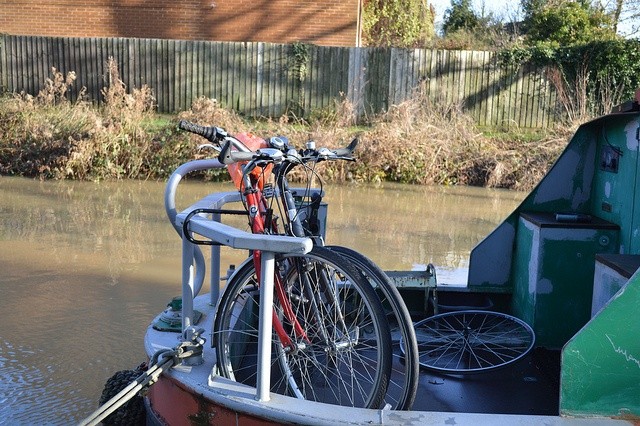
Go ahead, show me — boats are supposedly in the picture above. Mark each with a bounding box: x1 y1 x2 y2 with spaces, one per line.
97 88 640 425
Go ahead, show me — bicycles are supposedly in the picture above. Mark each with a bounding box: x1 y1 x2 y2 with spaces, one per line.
178 119 392 410
220 130 420 411
